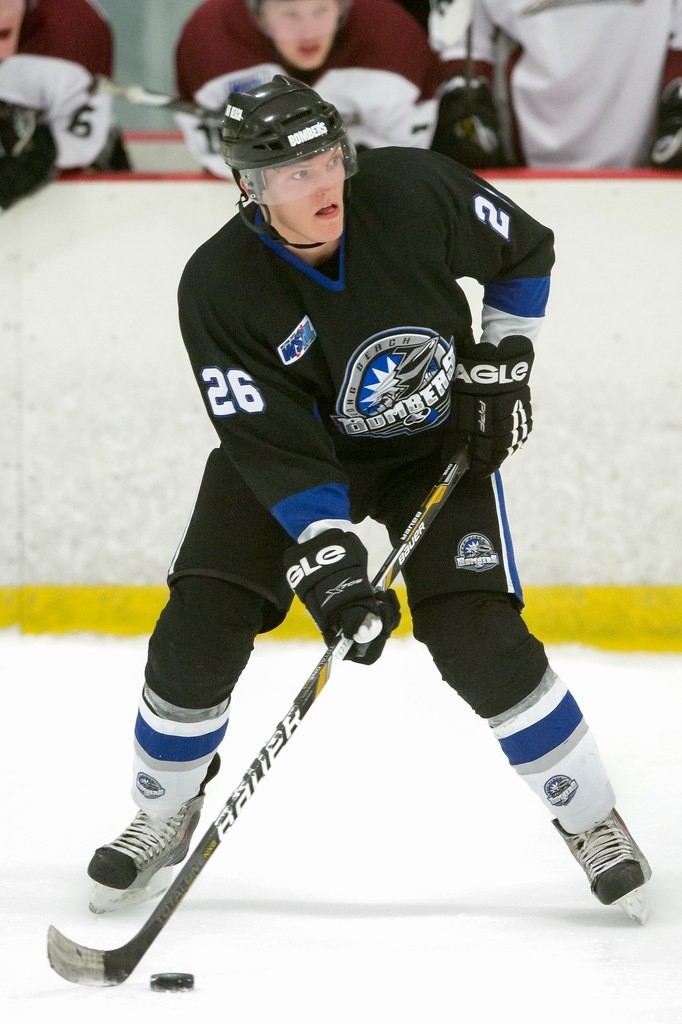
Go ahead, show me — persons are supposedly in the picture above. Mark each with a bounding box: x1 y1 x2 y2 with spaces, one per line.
174 0 682 180
0 0 131 209
74 77 652 923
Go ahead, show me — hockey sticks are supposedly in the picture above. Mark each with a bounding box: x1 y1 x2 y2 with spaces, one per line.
45 440 478 989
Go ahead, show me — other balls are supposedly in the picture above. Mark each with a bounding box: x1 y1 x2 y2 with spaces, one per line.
149 972 194 992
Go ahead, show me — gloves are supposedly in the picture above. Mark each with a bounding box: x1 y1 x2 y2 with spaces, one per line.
452 335 535 479
283 528 402 665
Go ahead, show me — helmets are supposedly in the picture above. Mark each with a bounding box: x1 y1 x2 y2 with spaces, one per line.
218 75 347 170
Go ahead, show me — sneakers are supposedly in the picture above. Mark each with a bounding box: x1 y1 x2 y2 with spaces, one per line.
551 808 656 925
87 752 221 912
